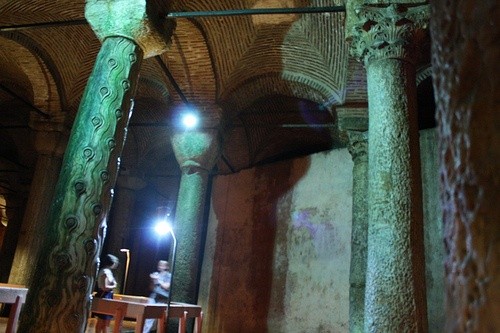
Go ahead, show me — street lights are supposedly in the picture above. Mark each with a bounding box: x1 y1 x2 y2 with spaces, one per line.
154 220 177 311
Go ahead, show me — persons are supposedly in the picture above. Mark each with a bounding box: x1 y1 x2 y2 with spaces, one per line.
143 259 171 333
93 253 116 332
